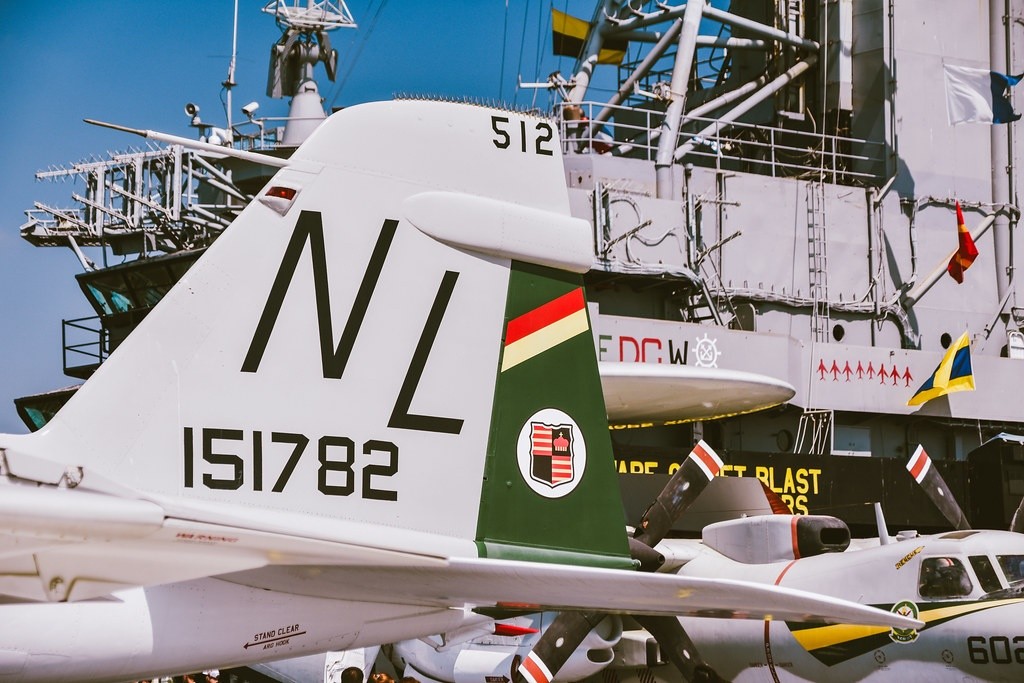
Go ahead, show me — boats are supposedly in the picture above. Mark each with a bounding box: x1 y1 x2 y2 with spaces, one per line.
13 0 1024 541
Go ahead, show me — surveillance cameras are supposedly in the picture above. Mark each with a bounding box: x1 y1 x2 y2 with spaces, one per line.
242 101 259 114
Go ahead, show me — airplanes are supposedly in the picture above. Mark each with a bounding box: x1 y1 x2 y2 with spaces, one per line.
0 102 1024 683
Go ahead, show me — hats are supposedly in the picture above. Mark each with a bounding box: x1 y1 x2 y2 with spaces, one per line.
203 668 220 678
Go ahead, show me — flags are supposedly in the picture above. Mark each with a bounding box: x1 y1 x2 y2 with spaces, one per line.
904 330 977 406
551 8 630 66
946 200 978 285
943 63 1024 128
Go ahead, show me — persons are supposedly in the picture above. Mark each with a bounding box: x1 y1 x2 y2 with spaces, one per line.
340 666 364 683
138 667 219 683
